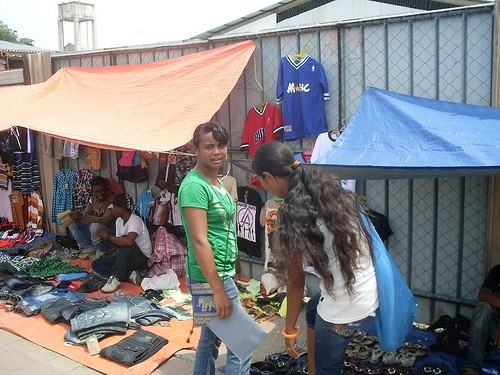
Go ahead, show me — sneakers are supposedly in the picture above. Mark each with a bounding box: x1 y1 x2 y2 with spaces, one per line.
101 276 121 293
129 270 143 285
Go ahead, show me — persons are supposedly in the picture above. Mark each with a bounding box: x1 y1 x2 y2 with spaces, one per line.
70 175 116 261
252 141 378 375
91 194 152 294
461 264 500 375
178 120 252 375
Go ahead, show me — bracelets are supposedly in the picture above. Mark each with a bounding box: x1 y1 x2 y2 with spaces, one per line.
106 233 112 242
282 325 300 338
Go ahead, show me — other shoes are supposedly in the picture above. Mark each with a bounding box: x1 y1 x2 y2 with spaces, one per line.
250 331 448 375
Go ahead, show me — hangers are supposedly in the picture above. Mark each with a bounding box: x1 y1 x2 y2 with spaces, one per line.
256 92 267 109
242 177 252 188
291 43 311 59
330 118 348 134
357 196 369 211
273 195 284 204
303 141 314 159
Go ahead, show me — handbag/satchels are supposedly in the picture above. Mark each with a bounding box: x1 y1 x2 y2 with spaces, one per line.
148 226 188 278
349 192 416 351
190 282 224 326
